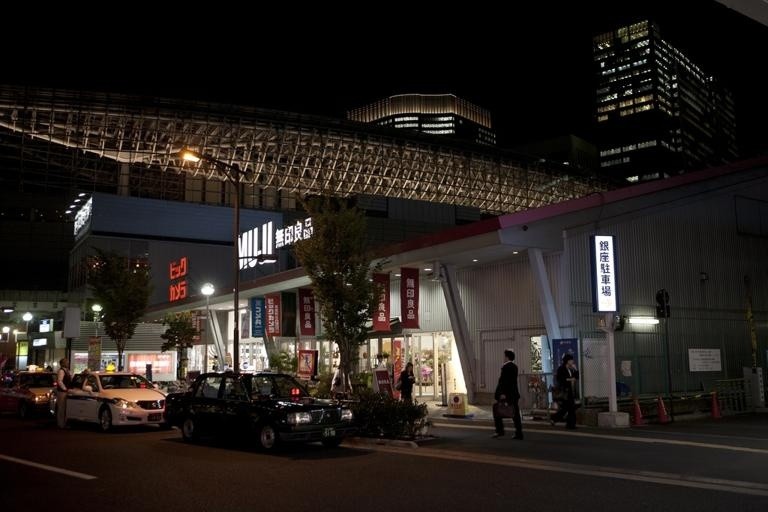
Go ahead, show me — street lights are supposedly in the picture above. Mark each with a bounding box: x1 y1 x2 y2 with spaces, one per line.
21 312 34 332
90 302 104 338
200 281 216 375
179 148 281 372
2 326 11 369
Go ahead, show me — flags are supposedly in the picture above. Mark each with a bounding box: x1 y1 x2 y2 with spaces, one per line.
401 268 420 329
263 294 282 336
250 297 266 338
280 291 298 338
297 287 316 336
372 273 391 332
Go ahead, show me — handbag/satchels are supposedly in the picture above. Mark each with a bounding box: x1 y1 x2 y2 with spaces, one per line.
493 398 514 418
552 386 569 401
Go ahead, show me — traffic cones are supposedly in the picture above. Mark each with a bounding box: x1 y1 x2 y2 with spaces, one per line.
708 385 726 420
654 393 676 425
629 399 649 427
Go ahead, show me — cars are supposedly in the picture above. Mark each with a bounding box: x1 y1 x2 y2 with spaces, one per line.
48 370 170 433
163 371 355 454
0 369 59 419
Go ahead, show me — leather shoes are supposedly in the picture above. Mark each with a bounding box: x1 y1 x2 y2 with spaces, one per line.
491 431 523 440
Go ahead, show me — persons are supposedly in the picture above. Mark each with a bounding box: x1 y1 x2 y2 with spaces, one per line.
549 354 582 429
376 353 387 368
398 363 416 402
56 357 71 427
491 348 523 441
330 365 354 400
